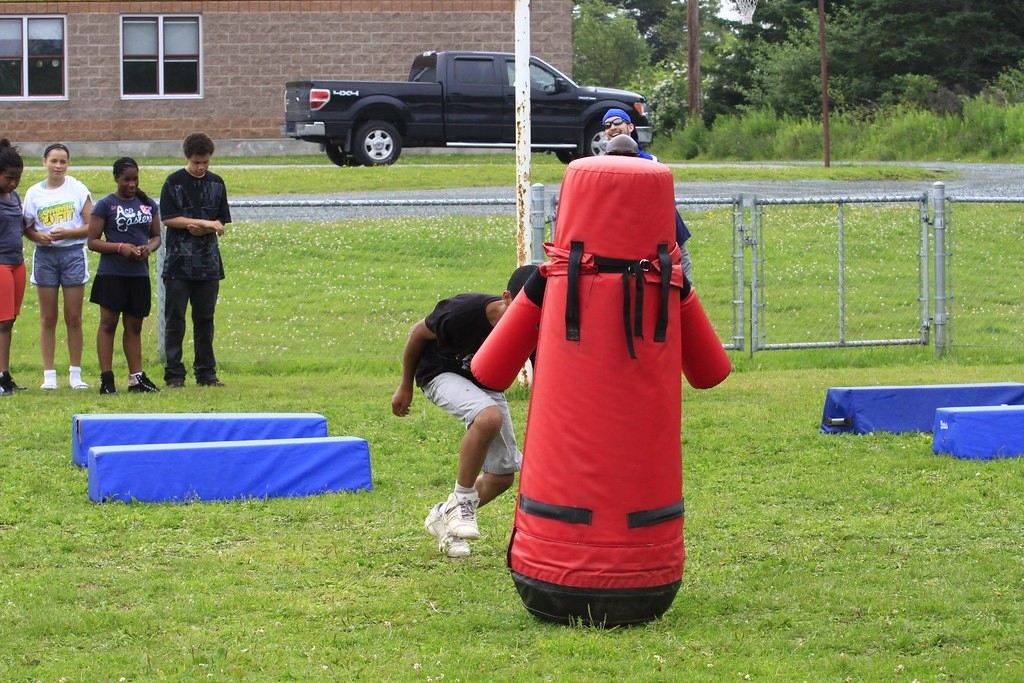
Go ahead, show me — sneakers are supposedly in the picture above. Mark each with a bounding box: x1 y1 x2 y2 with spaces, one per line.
128 371 158 392
0 371 13 395
439 493 480 539
99 370 116 395
425 502 470 557
11 378 28 390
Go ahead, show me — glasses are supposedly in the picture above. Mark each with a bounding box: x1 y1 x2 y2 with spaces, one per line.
605 151 639 157
603 118 628 129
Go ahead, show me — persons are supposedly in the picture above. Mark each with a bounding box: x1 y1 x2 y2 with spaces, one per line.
160 133 232 388
605 134 692 282
88 156 162 393
22 144 94 390
0 139 27 396
602 109 658 161
392 265 541 558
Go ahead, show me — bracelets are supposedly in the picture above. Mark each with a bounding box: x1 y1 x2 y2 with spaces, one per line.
117 243 123 252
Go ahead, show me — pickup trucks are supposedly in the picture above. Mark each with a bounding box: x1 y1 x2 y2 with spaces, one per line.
280 48 657 167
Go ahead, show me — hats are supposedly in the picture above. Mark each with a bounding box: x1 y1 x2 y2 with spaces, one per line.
602 108 639 141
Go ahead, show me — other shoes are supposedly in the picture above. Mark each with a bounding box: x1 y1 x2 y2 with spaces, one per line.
41 382 57 390
69 381 89 390
168 382 185 387
203 380 226 386
